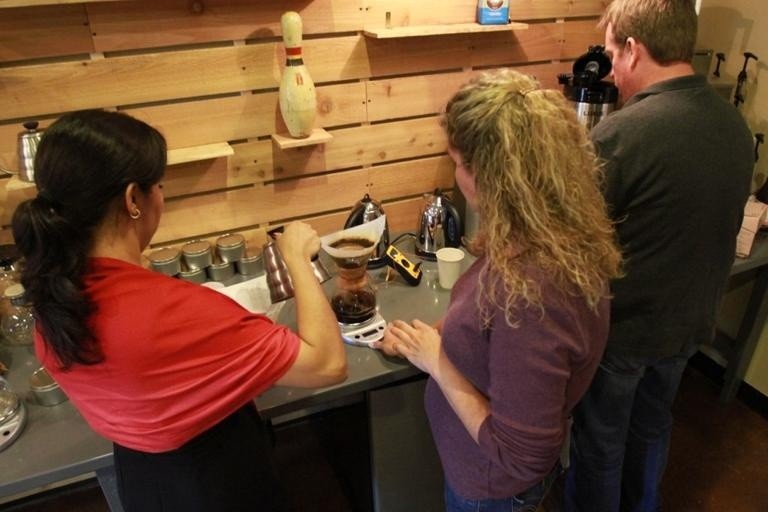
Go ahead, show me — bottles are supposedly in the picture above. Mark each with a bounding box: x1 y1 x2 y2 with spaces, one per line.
0 282 70 408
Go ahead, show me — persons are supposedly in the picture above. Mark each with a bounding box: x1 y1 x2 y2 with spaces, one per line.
10 108 348 512
372 66 630 510
561 0 756 511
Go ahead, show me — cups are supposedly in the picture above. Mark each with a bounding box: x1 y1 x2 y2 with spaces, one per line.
436 247 466 291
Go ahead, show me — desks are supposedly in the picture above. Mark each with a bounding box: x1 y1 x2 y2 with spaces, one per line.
2 223 768 512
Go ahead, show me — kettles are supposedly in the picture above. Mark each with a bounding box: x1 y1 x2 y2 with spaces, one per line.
341 193 392 271
415 188 467 263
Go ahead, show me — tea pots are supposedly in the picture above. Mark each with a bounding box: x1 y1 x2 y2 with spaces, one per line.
262 224 333 304
